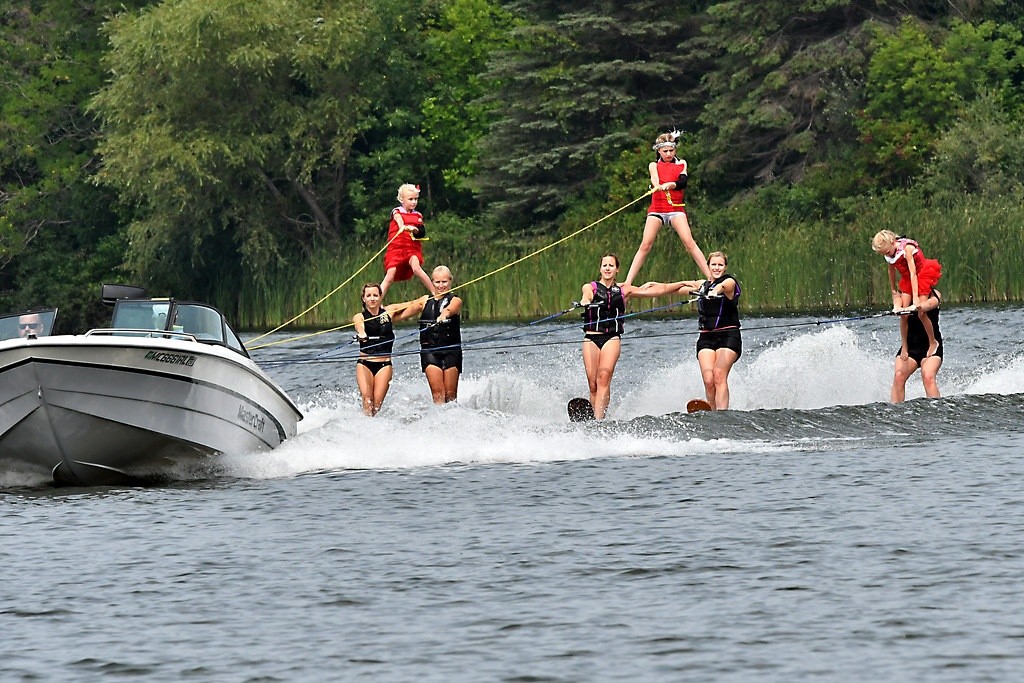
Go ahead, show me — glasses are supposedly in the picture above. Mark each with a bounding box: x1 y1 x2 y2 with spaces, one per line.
18 322 40 329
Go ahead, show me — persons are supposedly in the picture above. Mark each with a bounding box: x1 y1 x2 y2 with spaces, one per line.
379 182 439 303
354 284 428 416
638 252 741 414
612 132 715 284
581 254 695 421
873 227 943 400
389 265 466 404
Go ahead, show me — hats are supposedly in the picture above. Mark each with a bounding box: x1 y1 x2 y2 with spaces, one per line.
152 304 178 316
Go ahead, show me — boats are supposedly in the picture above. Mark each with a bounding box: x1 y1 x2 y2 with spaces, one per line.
0 283 303 488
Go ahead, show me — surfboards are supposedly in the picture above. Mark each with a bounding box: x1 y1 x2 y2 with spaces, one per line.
685 398 713 414
567 398 595 423
